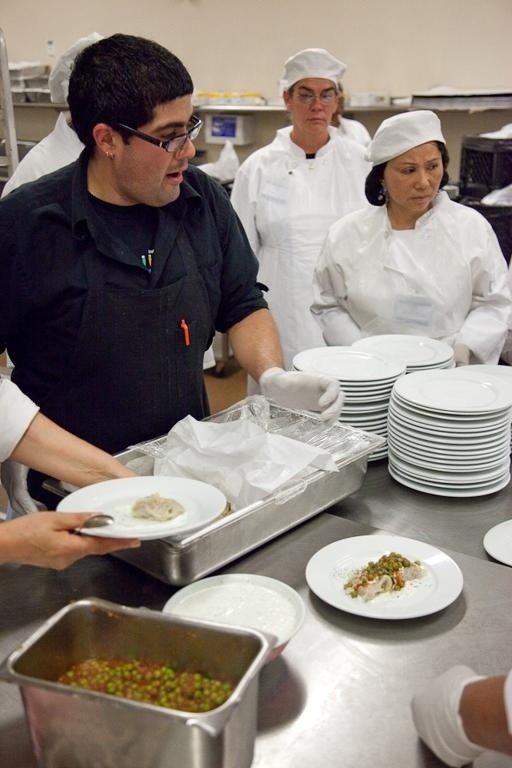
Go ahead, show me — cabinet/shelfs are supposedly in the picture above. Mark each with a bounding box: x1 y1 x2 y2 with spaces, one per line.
0 102 79 183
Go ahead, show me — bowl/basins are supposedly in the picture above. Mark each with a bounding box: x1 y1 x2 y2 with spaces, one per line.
7 64 52 103
162 572 309 667
442 184 459 199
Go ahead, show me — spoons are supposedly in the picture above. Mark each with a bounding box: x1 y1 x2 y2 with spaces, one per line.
70 515 116 536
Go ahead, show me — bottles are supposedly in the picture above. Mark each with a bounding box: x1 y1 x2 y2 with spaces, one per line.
195 91 262 106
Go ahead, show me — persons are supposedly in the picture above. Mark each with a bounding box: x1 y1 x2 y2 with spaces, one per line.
227 46 371 401
310 107 512 365
0 34 108 199
272 77 371 158
2 381 142 572
0 31 345 506
411 664 509 768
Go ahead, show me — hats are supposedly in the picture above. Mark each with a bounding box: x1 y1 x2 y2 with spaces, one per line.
281 48 347 93
363 109 447 167
47 31 105 107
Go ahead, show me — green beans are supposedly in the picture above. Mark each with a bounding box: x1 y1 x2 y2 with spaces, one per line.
57 657 234 713
353 551 420 593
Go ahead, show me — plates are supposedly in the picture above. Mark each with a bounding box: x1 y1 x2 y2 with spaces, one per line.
57 475 228 542
290 333 511 499
305 533 466 622
483 520 511 567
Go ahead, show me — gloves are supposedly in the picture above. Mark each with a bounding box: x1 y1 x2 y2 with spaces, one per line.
258 366 345 428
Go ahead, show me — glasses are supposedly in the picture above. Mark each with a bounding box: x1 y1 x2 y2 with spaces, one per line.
106 109 203 153
288 91 340 107
